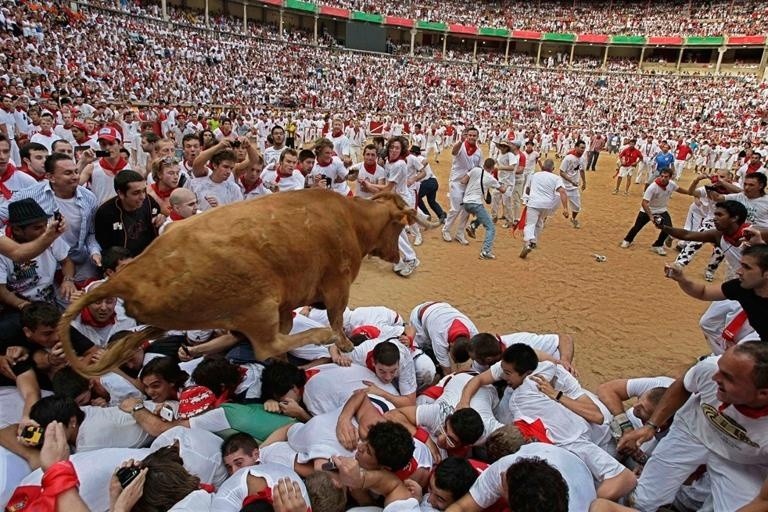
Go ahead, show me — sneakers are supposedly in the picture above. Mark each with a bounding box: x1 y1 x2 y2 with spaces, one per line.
611 189 713 281
392 217 579 276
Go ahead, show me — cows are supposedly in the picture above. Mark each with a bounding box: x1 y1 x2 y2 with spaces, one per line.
56 188 447 379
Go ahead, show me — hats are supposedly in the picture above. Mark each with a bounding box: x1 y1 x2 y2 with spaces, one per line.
177 386 215 420
492 140 511 149
97 127 121 144
8 197 53 226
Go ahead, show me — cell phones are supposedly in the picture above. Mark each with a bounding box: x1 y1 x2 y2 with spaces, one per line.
159 407 173 422
53 207 61 231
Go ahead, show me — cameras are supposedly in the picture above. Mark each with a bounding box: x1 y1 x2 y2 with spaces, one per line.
322 175 332 188
228 139 241 149
322 458 339 472
349 169 359 175
96 150 110 158
20 425 45 449
116 464 140 488
653 216 665 227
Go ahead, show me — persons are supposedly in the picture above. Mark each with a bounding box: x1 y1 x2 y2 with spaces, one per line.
0 1 768 512
